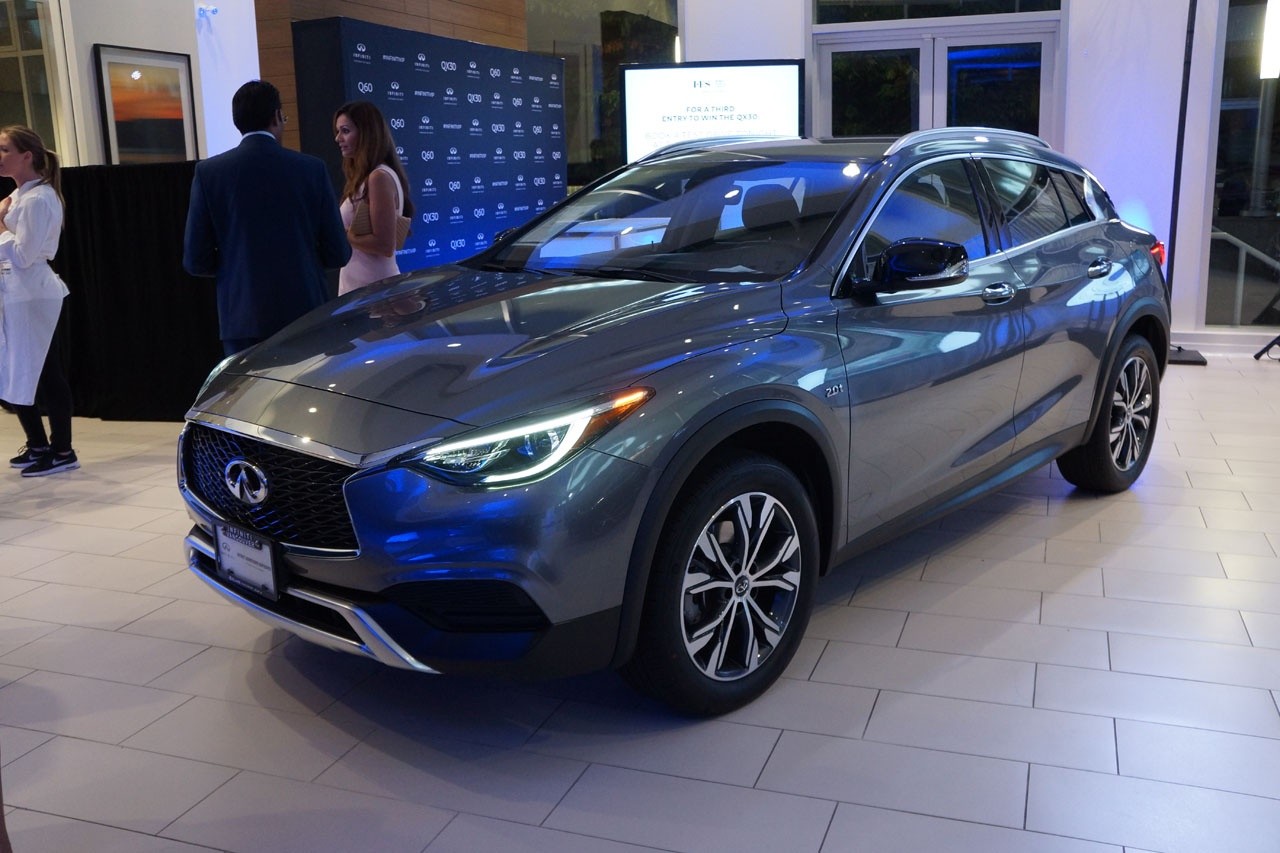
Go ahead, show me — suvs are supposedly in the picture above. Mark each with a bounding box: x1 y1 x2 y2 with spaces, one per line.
173 127 1176 720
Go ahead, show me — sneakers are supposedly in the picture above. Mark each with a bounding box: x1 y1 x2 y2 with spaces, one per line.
20 449 81 477
9 444 50 468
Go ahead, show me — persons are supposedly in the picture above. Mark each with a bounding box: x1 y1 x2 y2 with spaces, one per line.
183 81 354 356
334 100 416 296
1217 163 1246 216
0 125 80 477
590 139 605 161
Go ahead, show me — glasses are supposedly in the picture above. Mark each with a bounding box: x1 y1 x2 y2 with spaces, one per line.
279 107 289 124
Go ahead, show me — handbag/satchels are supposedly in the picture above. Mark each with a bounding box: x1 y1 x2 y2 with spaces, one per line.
348 198 411 250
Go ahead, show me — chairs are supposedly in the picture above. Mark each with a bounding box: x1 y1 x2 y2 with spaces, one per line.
725 185 802 251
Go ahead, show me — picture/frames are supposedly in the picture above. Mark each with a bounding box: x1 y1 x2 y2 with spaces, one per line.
93 43 200 164
